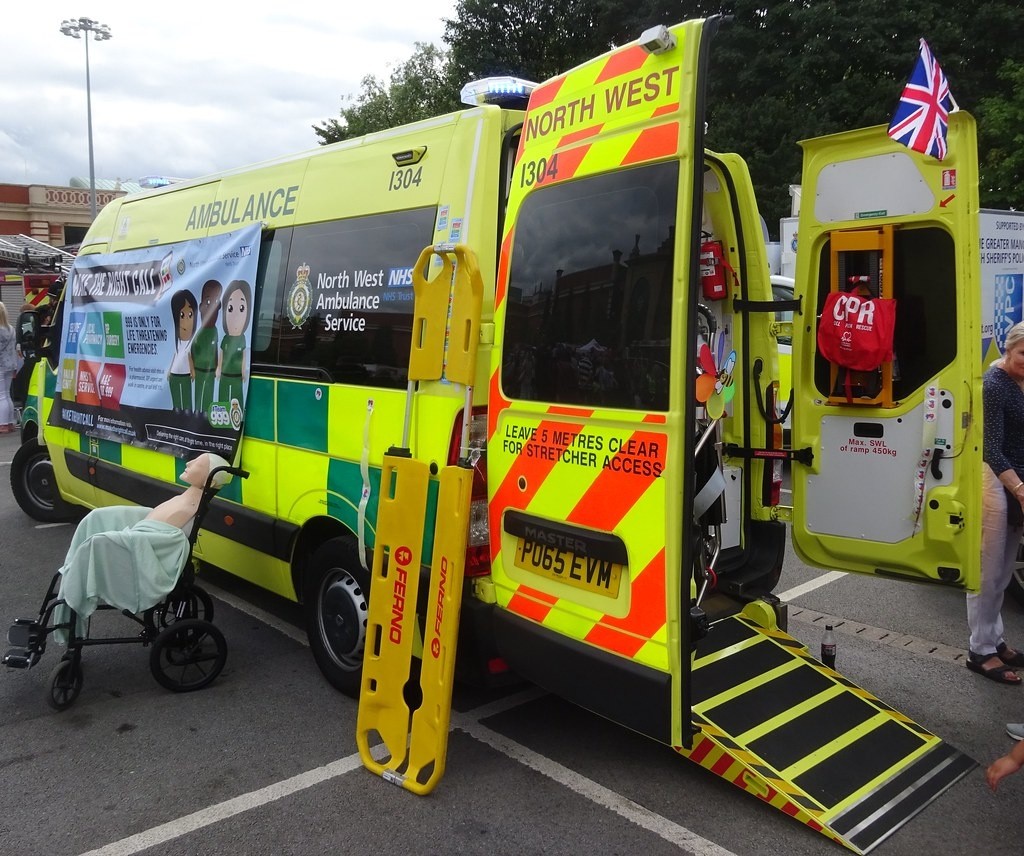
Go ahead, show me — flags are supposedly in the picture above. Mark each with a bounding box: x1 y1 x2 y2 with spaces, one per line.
887 43 960 161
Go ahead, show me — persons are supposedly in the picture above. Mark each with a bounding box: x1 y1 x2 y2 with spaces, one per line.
0 302 18 433
10 303 46 415
966 321 1024 684
134 453 233 533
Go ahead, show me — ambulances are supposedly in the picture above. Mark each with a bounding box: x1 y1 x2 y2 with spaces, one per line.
9 15 985 854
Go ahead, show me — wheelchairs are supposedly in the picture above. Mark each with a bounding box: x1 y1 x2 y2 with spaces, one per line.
2 467 251 714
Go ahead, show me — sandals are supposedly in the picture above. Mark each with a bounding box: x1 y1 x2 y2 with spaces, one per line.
966 647 1022 684
995 642 1024 665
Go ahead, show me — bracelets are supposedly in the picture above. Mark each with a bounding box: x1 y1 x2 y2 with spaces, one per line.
1012 482 1023 494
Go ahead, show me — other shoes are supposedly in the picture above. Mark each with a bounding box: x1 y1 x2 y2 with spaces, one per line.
0 424 15 433
